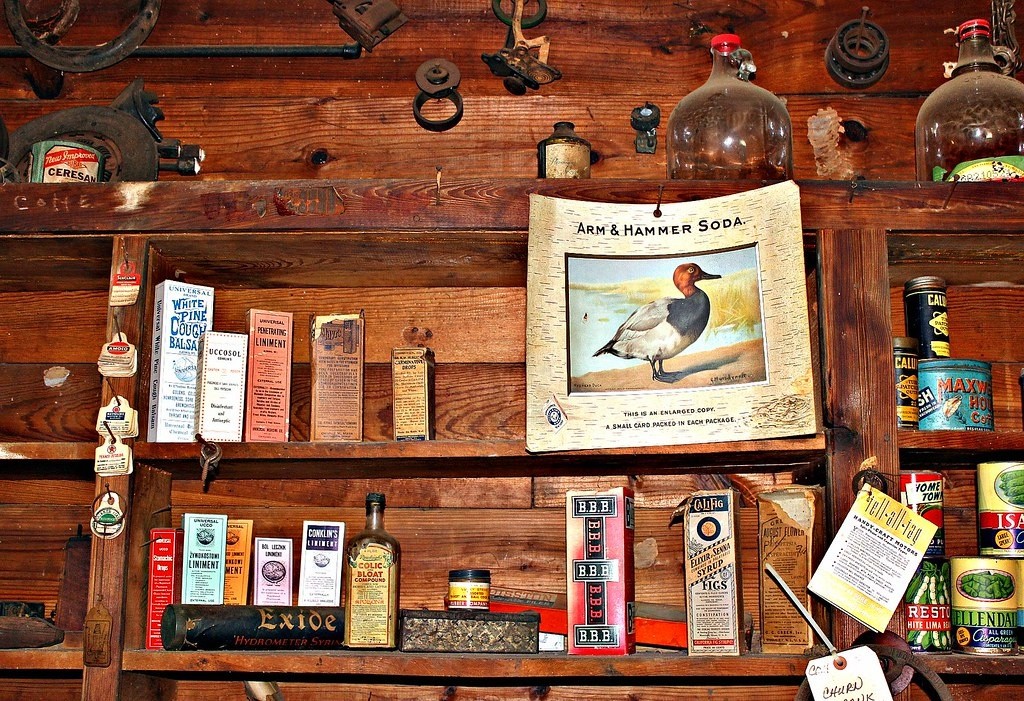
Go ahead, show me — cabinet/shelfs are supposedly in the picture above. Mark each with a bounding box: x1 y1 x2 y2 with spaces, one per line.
0 178 1024 701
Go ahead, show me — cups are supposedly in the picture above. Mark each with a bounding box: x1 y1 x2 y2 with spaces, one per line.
891 337 920 430
903 274 951 362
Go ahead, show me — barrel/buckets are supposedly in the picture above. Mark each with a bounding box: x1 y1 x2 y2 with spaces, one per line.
899 461 1024 656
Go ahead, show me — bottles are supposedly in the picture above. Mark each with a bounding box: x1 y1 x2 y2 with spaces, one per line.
537 121 592 178
915 20 1024 181
343 491 402 649
666 33 790 180
